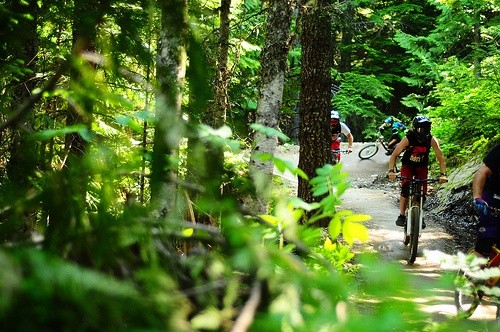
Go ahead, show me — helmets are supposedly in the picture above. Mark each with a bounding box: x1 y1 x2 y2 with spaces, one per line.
413 116 432 137
385 117 393 123
331 111 339 127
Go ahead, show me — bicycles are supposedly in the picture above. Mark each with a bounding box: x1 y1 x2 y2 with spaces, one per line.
452 201 500 320
358 127 406 160
331 149 351 166
386 168 449 266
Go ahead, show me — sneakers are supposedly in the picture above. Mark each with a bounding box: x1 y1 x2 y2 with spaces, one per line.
396 214 406 226
422 217 426 229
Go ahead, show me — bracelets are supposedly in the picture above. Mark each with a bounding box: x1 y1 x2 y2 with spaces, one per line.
441 172 446 176
388 169 394 173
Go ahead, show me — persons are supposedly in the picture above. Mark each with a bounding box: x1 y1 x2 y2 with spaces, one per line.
330 110 353 164
470 143 500 279
387 115 447 229
377 117 407 156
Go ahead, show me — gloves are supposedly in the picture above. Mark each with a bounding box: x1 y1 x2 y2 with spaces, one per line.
472 198 489 215
